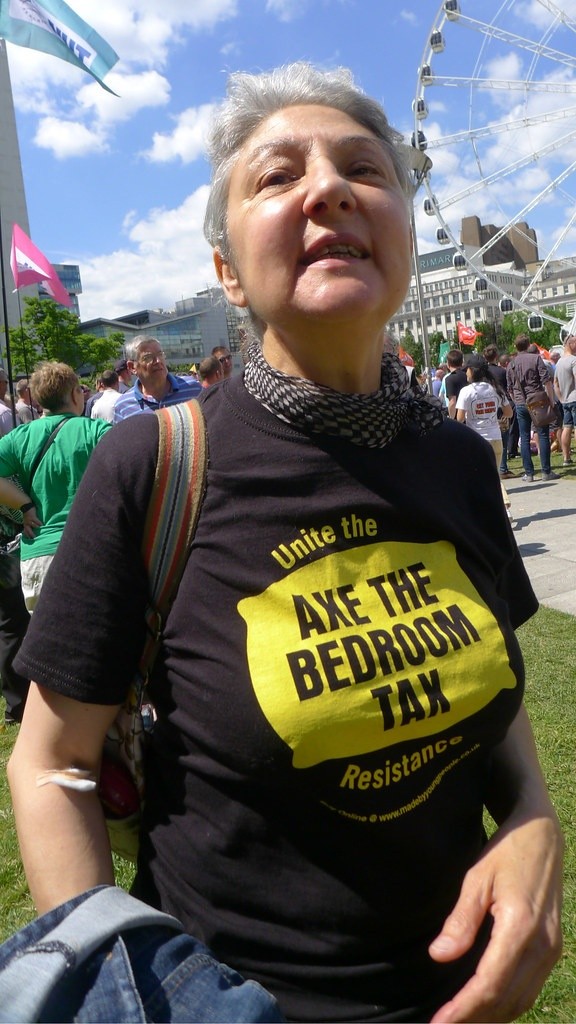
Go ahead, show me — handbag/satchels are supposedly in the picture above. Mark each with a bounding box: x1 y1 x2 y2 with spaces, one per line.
524 392 555 425
1 533 21 591
92 632 155 865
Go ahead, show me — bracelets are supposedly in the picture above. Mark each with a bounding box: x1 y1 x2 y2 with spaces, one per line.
20 502 34 513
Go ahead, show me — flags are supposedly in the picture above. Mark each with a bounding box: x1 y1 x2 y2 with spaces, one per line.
10 222 74 309
457 321 484 346
0 0 123 98
399 345 413 366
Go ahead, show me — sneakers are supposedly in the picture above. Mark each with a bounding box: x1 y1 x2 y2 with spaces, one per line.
542 472 562 480
522 474 534 482
563 460 576 466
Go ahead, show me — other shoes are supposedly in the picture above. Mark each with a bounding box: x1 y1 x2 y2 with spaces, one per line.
501 471 519 478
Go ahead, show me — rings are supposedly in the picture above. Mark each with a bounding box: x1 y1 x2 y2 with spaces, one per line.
32 522 34 524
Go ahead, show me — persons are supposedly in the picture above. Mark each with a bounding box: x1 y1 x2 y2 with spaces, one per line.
0 344 235 721
0 360 116 615
402 333 576 525
113 332 206 427
2 58 565 1023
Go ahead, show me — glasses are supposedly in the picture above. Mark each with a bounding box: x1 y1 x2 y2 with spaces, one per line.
219 354 233 363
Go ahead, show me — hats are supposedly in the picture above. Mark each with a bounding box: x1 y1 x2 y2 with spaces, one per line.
116 360 130 372
461 354 489 371
